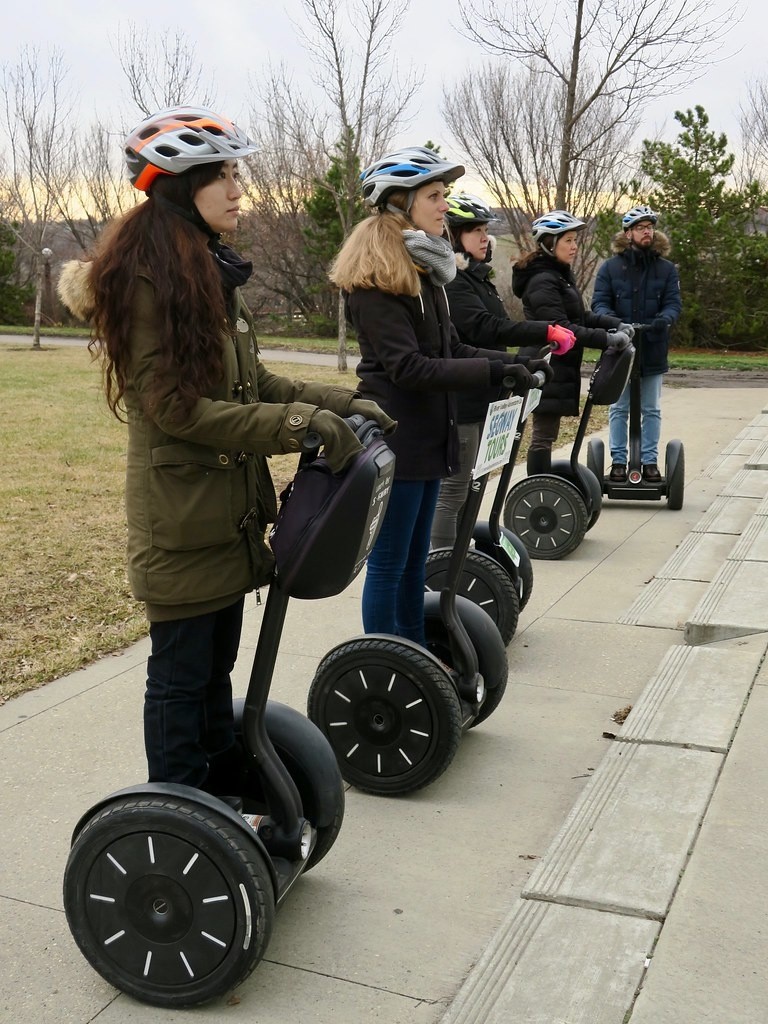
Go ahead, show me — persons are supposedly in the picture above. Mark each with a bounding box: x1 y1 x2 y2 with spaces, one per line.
332 146 554 674
431 193 576 553
589 204 686 483
511 210 635 475
56 103 399 817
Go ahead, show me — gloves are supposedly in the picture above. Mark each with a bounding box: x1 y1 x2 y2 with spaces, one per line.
607 331 630 350
504 362 534 398
527 358 554 389
618 322 635 339
547 323 577 355
652 317 668 333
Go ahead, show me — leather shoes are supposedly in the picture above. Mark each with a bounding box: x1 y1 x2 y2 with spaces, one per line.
643 464 661 482
609 463 627 482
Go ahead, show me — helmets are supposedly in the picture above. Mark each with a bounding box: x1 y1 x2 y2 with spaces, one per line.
359 146 466 206
442 196 501 224
531 209 589 242
121 103 263 190
621 205 658 233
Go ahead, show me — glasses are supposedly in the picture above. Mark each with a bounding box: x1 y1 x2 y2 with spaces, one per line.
631 222 654 233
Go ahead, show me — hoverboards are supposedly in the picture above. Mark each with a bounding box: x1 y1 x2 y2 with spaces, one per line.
503 319 650 561
304 363 508 802
421 334 555 647
60 412 397 1010
587 320 686 510
